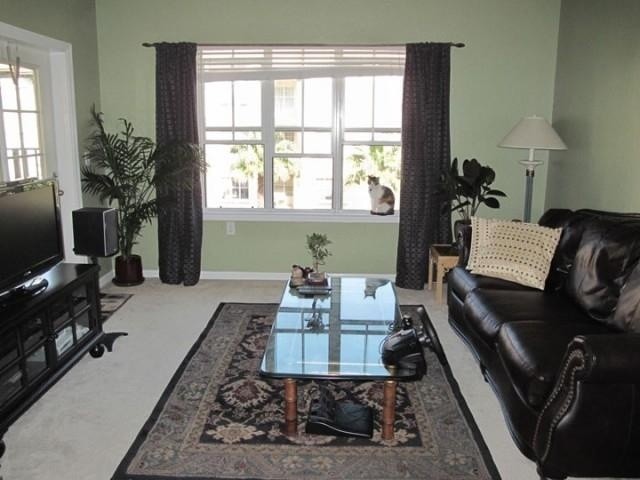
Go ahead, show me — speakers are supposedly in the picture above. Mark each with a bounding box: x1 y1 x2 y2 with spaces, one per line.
72 207 120 257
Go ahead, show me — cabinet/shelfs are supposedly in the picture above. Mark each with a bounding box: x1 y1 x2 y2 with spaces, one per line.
1 263 106 458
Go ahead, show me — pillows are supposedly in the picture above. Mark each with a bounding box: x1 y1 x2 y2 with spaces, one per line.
465 215 563 292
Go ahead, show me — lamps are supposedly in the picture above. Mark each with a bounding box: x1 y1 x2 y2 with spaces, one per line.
498 115 568 223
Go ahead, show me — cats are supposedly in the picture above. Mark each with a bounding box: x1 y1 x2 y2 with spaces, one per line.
368 176 395 215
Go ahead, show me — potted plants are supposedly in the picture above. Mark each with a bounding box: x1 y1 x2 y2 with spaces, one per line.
78 101 211 285
304 232 333 283
430 157 509 253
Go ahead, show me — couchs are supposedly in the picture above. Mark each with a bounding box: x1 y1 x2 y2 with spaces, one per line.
447 207 640 480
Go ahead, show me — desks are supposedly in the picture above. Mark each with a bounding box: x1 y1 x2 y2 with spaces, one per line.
428 244 461 304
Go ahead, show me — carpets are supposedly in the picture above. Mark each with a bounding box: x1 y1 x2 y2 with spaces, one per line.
109 302 503 480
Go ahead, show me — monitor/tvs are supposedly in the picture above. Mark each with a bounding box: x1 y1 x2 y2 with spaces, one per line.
0 178 64 307
